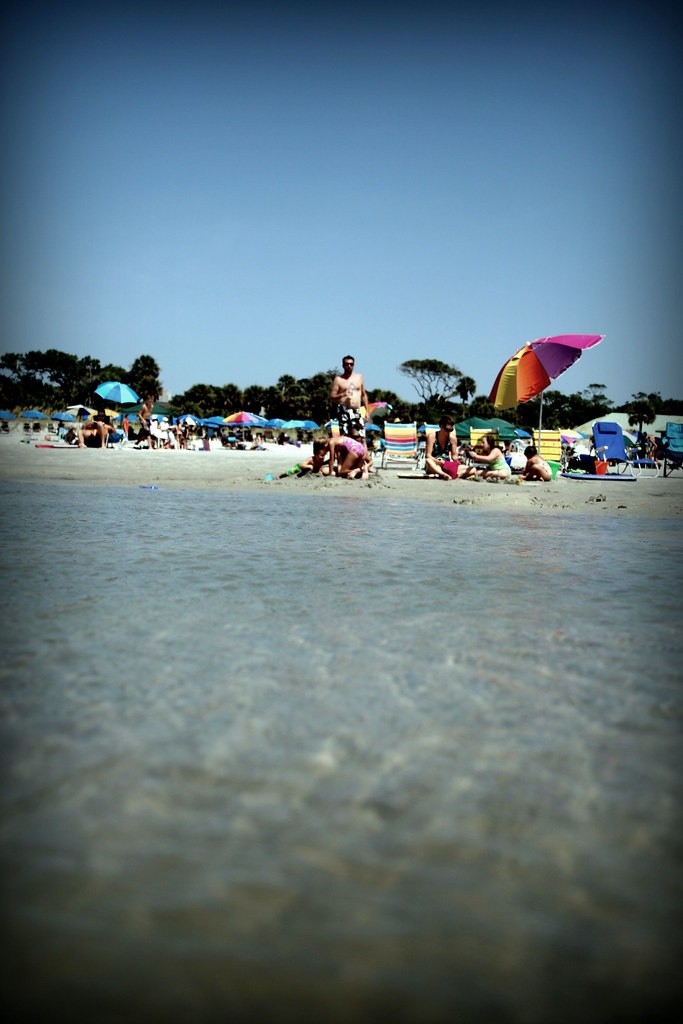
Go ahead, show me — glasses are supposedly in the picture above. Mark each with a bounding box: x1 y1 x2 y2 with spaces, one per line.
443 426 454 432
344 362 355 366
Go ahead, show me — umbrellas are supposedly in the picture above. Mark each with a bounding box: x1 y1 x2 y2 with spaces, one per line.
556 428 588 463
360 401 390 421
452 416 531 441
0 380 319 433
487 334 606 455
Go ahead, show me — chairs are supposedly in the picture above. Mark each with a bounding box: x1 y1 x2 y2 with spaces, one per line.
662 422 683 477
381 421 420 470
466 426 500 468
592 421 660 479
0 422 279 450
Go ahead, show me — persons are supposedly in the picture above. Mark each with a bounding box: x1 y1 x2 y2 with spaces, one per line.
121 413 129 446
643 431 665 478
298 436 373 479
468 435 512 480
523 445 552 481
137 393 155 449
561 442 581 472
149 415 305 450
423 416 477 481
79 409 123 448
331 355 376 473
